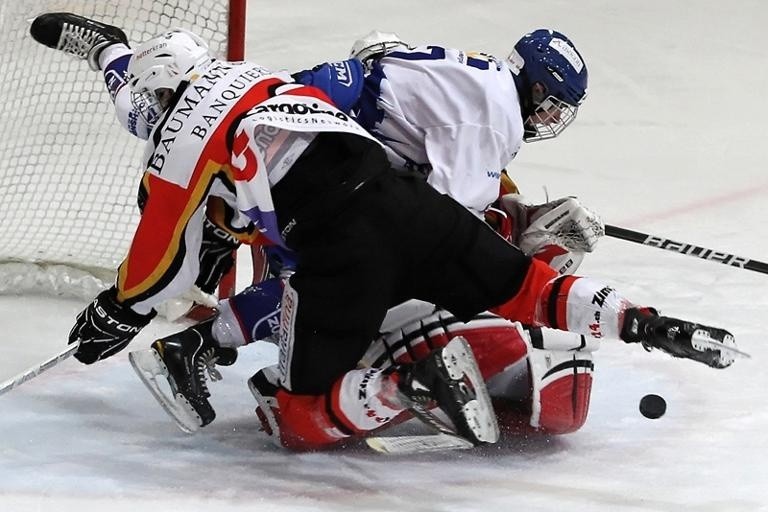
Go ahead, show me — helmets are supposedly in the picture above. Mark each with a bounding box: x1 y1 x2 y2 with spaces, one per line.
503 27 591 118
347 28 419 64
125 27 213 98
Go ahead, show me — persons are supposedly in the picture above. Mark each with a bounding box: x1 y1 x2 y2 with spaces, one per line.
67 27 736 452
343 28 609 435
28 12 590 426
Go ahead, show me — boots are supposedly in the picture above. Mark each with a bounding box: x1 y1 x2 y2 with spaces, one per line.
618 304 754 371
393 333 503 446
127 311 238 435
28 11 133 72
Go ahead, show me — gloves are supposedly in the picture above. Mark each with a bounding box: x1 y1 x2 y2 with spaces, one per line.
65 282 158 368
193 194 260 296
